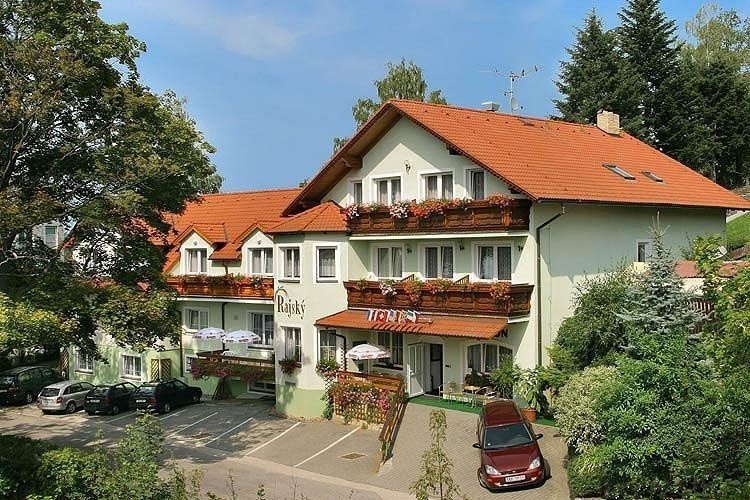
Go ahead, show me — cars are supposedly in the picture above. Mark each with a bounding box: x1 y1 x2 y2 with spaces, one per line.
37 379 97 415
81 381 141 416
473 397 553 493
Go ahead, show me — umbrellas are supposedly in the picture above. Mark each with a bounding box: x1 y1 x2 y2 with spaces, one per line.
192 326 227 354
221 329 261 357
345 341 391 375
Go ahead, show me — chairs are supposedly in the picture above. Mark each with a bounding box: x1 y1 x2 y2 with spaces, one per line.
220 351 235 363
438 380 501 409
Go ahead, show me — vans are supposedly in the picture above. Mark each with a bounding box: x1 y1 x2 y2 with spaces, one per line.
0 365 66 408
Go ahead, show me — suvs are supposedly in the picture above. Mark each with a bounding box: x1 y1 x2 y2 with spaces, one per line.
125 378 204 414
